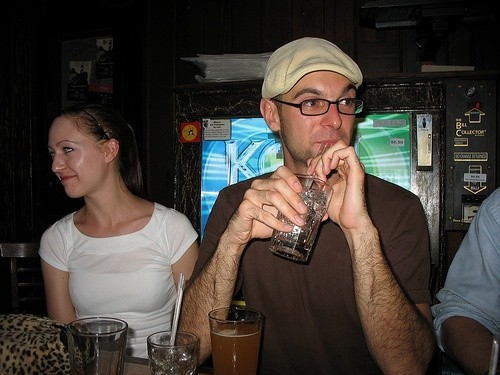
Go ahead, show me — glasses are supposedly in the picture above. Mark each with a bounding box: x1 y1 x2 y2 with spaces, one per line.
270 96 364 116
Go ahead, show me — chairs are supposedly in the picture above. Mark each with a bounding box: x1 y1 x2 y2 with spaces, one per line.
0 241 48 318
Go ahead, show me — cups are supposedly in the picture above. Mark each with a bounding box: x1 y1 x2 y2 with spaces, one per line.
147 331 198 375
208 307 263 375
268 173 334 262
67 317 128 375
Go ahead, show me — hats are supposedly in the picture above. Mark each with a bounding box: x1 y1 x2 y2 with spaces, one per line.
261 36 363 99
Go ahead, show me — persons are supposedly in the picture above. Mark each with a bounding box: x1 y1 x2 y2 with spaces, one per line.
39 104 198 358
178 37 434 375
431 187 500 375
